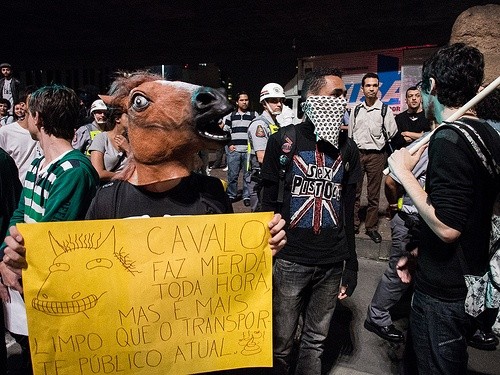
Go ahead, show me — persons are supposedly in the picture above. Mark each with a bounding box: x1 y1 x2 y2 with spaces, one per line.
364 130 500 347
0 85 101 352
72 99 109 161
0 146 23 248
258 63 361 375
6 100 25 125
389 87 431 153
210 87 234 172
348 73 398 243
222 90 260 206
248 83 285 212
88 106 130 184
387 42 500 375
0 98 14 127
0 86 43 188
0 69 287 269
0 63 22 116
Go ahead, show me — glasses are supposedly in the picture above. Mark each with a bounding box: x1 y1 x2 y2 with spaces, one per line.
415 80 424 92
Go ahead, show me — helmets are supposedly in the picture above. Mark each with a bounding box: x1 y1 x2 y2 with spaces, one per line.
89 99 108 116
260 84 286 103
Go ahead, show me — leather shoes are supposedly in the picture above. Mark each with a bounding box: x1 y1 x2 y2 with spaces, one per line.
462 323 500 350
363 318 405 342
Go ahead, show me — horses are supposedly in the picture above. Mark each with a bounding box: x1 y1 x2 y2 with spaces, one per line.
98 68 233 188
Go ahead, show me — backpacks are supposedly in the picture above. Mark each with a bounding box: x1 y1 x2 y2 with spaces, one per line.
463 213 500 339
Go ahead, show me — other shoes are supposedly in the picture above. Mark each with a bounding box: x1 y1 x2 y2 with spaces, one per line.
210 164 222 169
366 231 382 244
244 199 251 207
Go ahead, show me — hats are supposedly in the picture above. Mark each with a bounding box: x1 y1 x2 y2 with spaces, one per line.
1 62 12 68
